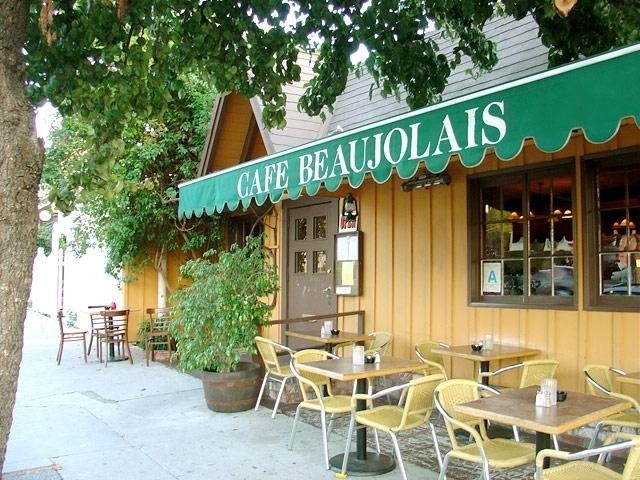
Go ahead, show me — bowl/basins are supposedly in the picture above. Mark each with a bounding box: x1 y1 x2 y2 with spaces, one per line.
331 330 340 334
471 344 483 351
557 391 567 401
365 356 375 362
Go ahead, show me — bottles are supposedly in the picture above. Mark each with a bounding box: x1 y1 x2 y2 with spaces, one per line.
535 391 543 407
320 325 325 334
544 390 551 408
375 351 382 363
479 340 483 346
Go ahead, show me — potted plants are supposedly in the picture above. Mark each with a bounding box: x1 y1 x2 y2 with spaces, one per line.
164 231 283 414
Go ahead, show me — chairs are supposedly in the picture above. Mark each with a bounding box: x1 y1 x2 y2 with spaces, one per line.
54 304 175 369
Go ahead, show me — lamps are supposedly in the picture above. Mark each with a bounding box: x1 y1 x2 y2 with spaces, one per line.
341 184 357 222
